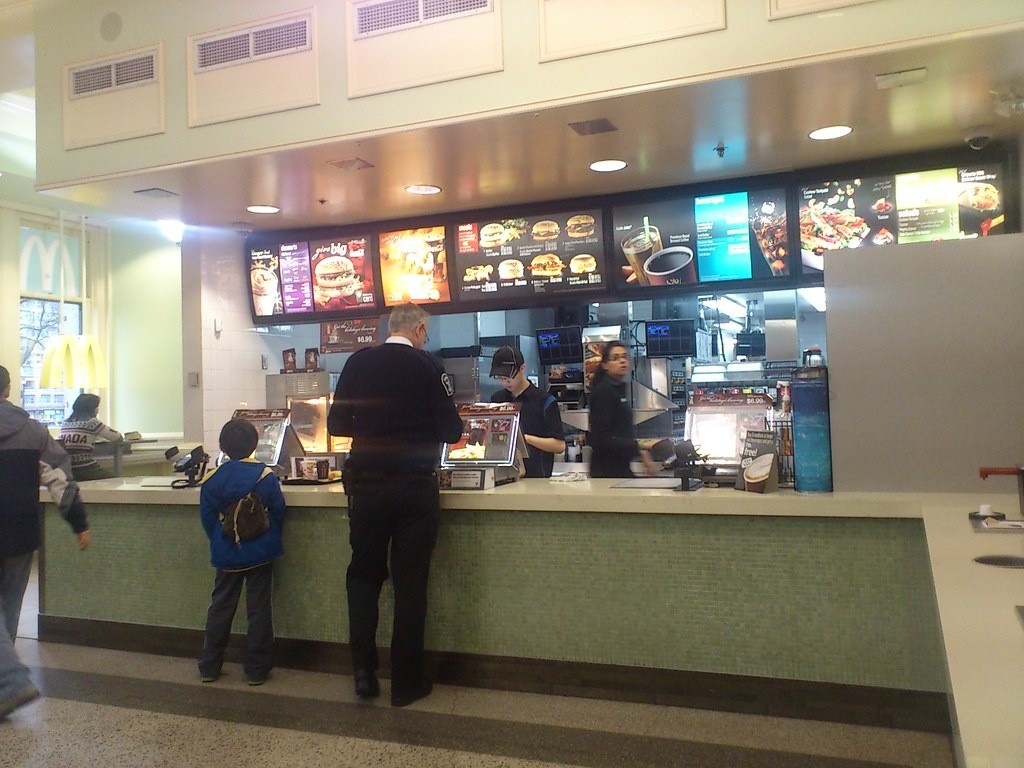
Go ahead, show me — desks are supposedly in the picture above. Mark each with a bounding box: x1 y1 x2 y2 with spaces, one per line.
921 492 1024 767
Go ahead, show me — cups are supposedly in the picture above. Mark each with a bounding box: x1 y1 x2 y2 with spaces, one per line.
621 225 664 286
643 245 697 286
316 460 329 479
250 267 277 315
744 452 774 493
302 461 315 479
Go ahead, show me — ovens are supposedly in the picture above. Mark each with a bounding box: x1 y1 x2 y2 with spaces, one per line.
266 370 352 454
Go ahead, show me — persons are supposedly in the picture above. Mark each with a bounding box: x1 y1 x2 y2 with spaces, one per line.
490 346 566 478
0 364 93 719
327 300 465 705
58 393 123 481
589 340 662 479
196 418 287 688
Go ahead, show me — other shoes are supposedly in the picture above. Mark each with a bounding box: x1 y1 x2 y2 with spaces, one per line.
390 680 432 707
200 670 217 681
0 679 39 719
247 675 264 684
354 670 378 695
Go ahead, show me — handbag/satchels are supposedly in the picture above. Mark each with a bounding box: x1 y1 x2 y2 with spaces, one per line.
221 491 270 546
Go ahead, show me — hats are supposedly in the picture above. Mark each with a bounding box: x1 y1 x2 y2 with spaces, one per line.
489 347 523 377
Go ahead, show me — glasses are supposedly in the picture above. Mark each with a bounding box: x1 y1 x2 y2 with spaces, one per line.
608 353 628 360
419 321 430 344
494 365 520 382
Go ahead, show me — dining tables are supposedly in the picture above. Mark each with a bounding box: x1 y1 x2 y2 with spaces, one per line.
54 438 157 478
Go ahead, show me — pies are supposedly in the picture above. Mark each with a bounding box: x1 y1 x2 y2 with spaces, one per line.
957 182 1000 212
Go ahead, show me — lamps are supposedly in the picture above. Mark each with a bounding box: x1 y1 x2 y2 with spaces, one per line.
808 125 854 140
589 159 628 172
39 333 100 388
246 205 280 214
406 184 442 195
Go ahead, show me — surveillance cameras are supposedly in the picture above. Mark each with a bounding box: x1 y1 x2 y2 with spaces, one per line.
232 222 254 238
964 128 994 149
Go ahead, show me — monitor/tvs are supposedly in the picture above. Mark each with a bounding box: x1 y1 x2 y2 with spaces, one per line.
536 325 583 366
736 334 765 356
645 319 696 359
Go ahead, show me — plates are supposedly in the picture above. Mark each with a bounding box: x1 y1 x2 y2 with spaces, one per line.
801 227 872 271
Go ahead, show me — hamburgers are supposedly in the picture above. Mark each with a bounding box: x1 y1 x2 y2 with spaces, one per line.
532 220 561 240
498 259 524 279
315 255 359 298
479 223 506 247
565 215 595 237
527 254 567 276
570 254 597 273
